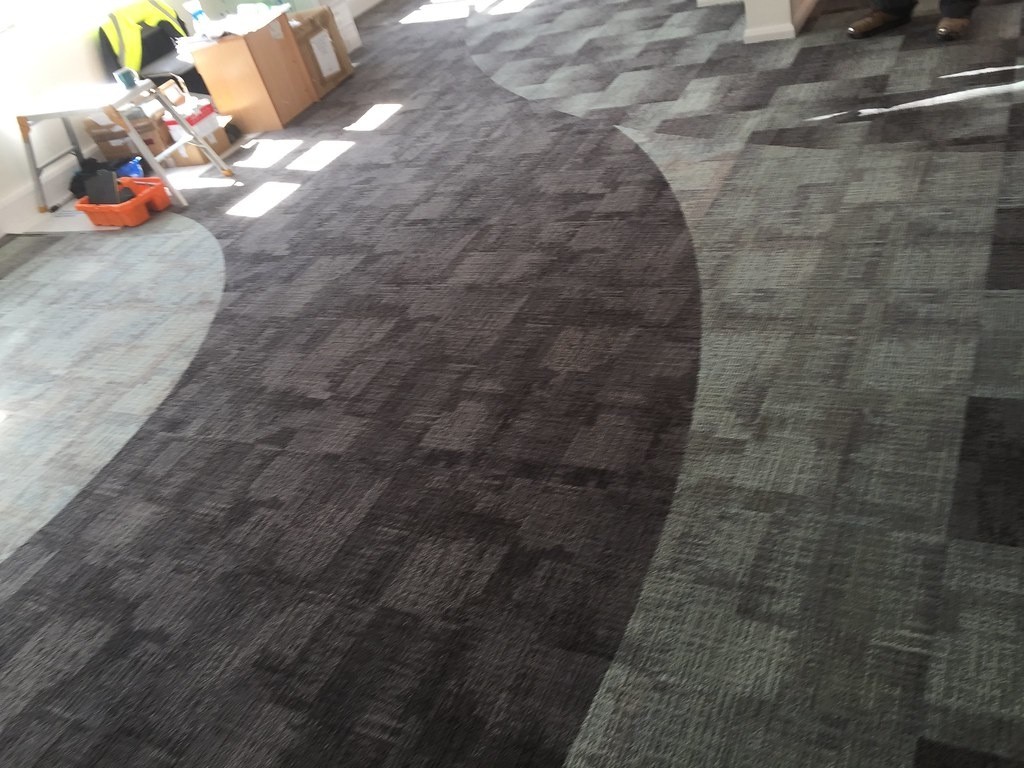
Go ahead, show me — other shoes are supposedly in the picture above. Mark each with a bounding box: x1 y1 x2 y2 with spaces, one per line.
847 8 911 39
934 15 972 41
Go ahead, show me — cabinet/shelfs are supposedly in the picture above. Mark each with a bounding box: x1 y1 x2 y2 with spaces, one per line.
189 12 321 131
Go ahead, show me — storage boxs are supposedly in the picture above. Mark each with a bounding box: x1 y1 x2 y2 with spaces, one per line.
75 177 171 226
287 6 356 99
84 80 184 168
166 115 233 166
160 99 219 140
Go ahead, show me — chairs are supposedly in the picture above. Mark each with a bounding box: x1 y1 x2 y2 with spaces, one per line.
99 0 209 95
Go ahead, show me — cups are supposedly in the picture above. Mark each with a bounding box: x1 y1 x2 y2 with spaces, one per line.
112 68 138 90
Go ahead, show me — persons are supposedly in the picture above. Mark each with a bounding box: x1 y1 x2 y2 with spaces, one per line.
845 0 981 41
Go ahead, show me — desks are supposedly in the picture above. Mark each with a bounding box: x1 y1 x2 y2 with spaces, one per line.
16 79 233 211
